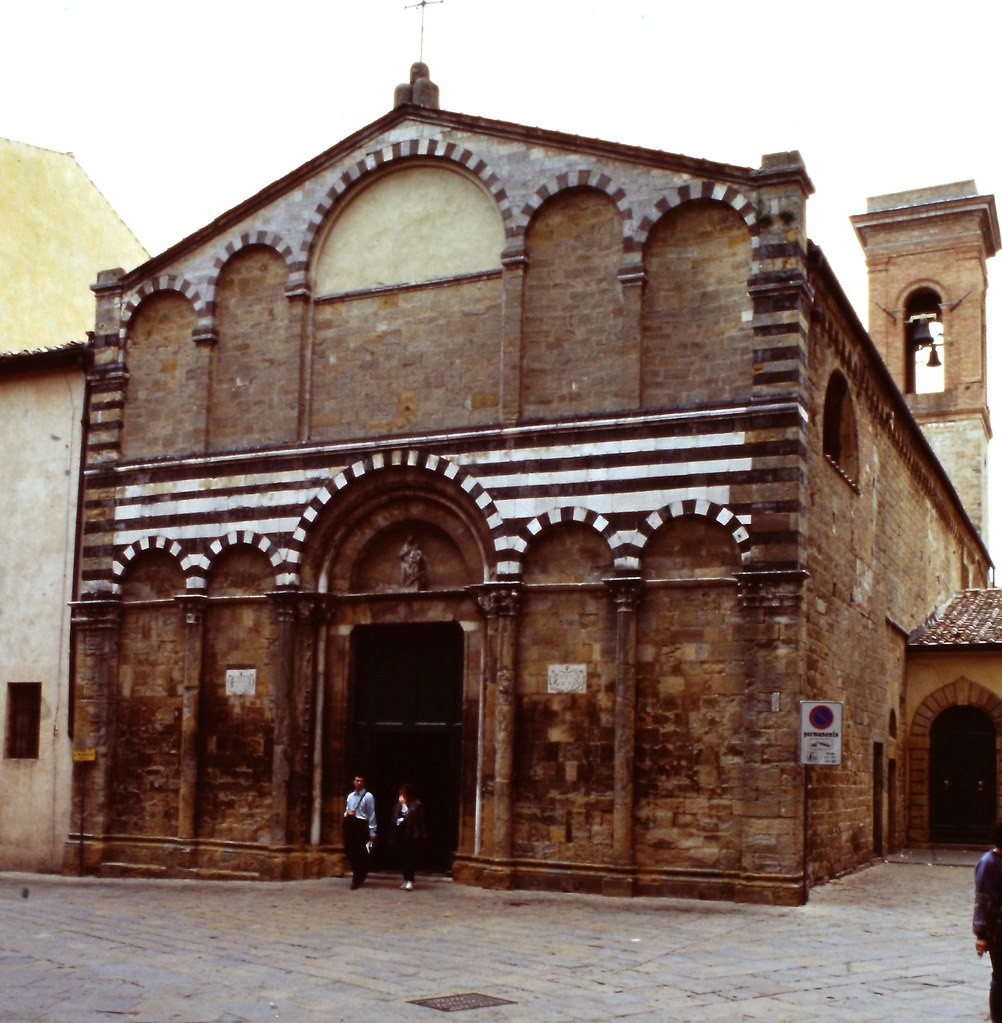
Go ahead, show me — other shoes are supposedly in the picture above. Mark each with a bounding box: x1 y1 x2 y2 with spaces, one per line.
350 882 360 890
400 882 406 889
406 880 413 890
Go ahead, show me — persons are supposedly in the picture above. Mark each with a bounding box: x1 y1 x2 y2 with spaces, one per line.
393 785 423 891
343 775 377 890
970 826 1002 1023
